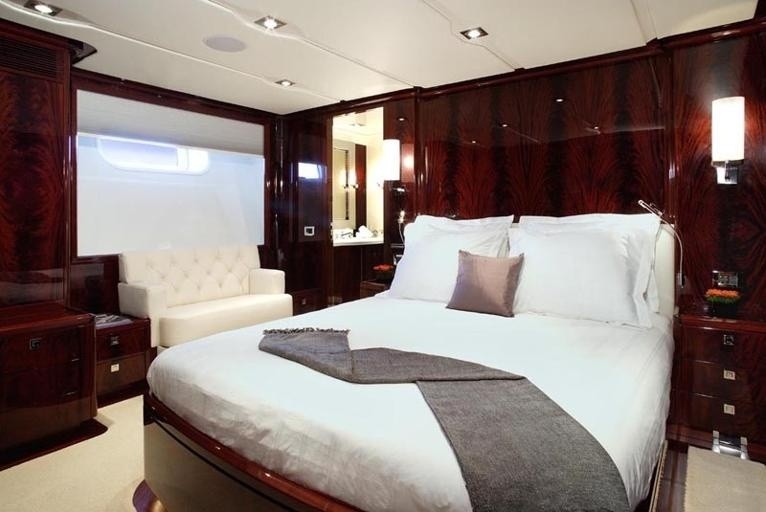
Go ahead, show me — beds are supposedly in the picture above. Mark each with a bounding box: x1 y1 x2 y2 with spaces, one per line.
133 212 675 512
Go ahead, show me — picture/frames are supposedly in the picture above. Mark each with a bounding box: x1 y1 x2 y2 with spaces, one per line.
712 271 743 290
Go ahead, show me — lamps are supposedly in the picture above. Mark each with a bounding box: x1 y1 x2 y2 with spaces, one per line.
638 199 685 289
378 139 407 194
397 210 406 246
712 97 745 186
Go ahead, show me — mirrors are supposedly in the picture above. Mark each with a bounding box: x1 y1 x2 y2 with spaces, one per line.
333 149 348 220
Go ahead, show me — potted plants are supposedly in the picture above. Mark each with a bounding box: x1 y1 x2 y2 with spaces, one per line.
706 290 742 318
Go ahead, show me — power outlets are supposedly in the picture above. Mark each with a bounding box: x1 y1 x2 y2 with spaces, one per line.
393 256 402 264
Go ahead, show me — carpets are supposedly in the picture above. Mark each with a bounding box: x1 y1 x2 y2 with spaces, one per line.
684 445 766 512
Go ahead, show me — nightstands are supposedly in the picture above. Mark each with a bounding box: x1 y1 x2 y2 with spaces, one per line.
359 279 387 298
668 316 766 462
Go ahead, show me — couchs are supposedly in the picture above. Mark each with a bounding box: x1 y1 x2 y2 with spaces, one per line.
118 245 292 354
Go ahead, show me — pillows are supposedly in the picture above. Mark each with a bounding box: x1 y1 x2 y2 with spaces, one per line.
519 213 662 314
508 224 653 328
391 234 514 300
456 247 520 315
390 214 514 302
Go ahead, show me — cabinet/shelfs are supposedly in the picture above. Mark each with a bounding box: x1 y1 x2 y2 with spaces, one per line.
292 286 323 317
70 254 151 396
0 21 98 449
